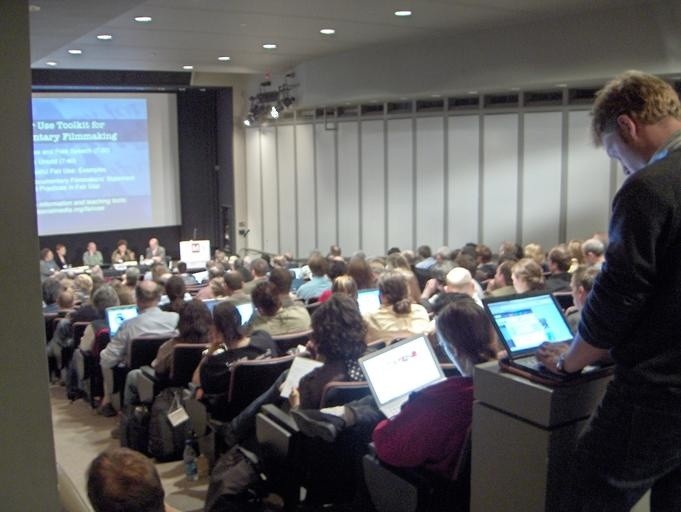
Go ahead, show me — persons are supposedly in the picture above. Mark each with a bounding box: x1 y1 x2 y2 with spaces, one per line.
159 276 191 314
282 253 297 265
69 285 121 401
363 271 430 345
432 242 497 268
473 262 500 290
374 300 496 481
111 299 215 439
326 246 346 280
433 291 476 317
241 258 271 294
444 268 475 297
247 282 313 337
42 270 94 321
40 248 60 275
483 260 517 296
368 262 386 278
380 252 421 305
206 247 241 280
301 250 321 280
347 256 380 289
497 241 524 265
591 230 609 245
95 280 180 417
544 248 573 293
417 261 461 314
228 255 254 283
147 301 288 460
176 263 199 285
415 244 437 270
143 262 168 286
567 242 581 274
87 448 167 512
112 239 134 264
514 258 549 293
243 267 308 326
84 241 104 266
586 240 606 270
54 243 72 268
403 252 431 298
536 71 681 512
296 253 334 301
219 272 253 307
524 243 548 272
270 255 292 294
563 266 600 337
110 267 139 304
194 266 228 299
208 299 368 447
141 239 166 266
306 275 358 362
261 254 276 275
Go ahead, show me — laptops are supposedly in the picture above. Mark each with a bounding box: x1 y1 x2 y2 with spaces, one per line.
104 304 141 336
357 289 381 315
358 332 448 421
482 290 600 388
236 302 257 326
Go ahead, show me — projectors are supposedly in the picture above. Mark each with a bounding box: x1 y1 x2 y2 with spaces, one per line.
243 73 300 129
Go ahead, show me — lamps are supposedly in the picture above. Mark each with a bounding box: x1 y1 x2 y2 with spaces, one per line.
243 81 279 127
270 71 300 119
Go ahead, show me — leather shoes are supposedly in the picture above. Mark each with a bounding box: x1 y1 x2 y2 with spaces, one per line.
97 404 117 417
112 428 122 439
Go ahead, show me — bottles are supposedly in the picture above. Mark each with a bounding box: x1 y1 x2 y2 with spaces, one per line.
140 255 144 262
183 441 199 482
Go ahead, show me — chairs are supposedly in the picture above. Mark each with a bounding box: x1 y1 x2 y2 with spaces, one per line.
61 322 87 370
136 343 207 407
45 313 65 382
78 329 110 408
361 428 470 512
238 381 370 512
185 355 296 476
270 330 312 356
113 337 170 409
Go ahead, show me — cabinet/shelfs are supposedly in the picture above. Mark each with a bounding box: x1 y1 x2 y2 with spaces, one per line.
471 359 614 512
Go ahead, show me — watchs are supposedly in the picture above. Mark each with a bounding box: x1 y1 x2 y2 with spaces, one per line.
554 353 585 375
